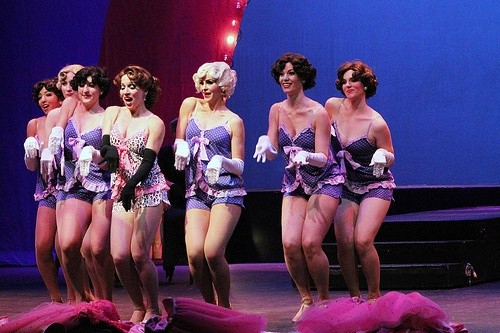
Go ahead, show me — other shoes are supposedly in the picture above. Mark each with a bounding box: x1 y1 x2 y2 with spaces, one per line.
291 298 315 323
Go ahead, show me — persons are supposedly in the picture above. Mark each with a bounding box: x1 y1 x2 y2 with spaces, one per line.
253 53 346 324
23 63 113 304
323 60 397 302
97 64 171 324
173 61 247 311
157 117 194 285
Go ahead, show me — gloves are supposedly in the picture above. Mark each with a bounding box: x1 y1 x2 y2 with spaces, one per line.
253 135 277 163
97 133 120 173
23 136 40 159
368 148 394 178
284 151 328 170
204 154 245 185
48 127 65 155
77 146 107 177
117 148 157 212
40 148 57 176
173 138 191 171
